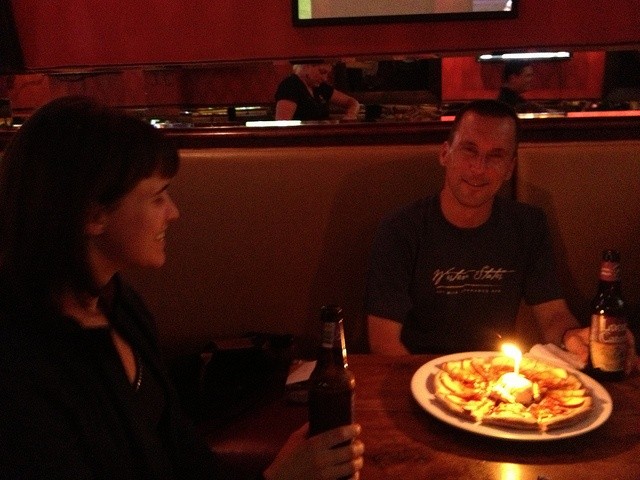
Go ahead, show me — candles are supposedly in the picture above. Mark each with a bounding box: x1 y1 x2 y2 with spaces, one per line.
500 342 525 376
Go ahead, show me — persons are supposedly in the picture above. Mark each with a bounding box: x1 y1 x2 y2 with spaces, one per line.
0 95 367 479
365 100 634 370
274 63 360 120
497 61 549 111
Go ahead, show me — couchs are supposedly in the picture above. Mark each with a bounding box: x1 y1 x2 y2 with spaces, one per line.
148 142 640 468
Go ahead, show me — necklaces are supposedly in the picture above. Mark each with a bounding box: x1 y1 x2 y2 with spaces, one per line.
133 361 142 395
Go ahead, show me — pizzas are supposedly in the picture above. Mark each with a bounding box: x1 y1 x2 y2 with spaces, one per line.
434 355 593 431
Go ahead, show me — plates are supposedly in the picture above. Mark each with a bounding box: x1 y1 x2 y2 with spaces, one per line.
410 350 614 442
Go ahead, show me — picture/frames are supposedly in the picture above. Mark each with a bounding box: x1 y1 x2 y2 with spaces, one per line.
292 0 520 28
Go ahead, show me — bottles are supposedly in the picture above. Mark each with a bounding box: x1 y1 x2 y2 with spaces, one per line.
308 303 357 446
589 250 629 380
